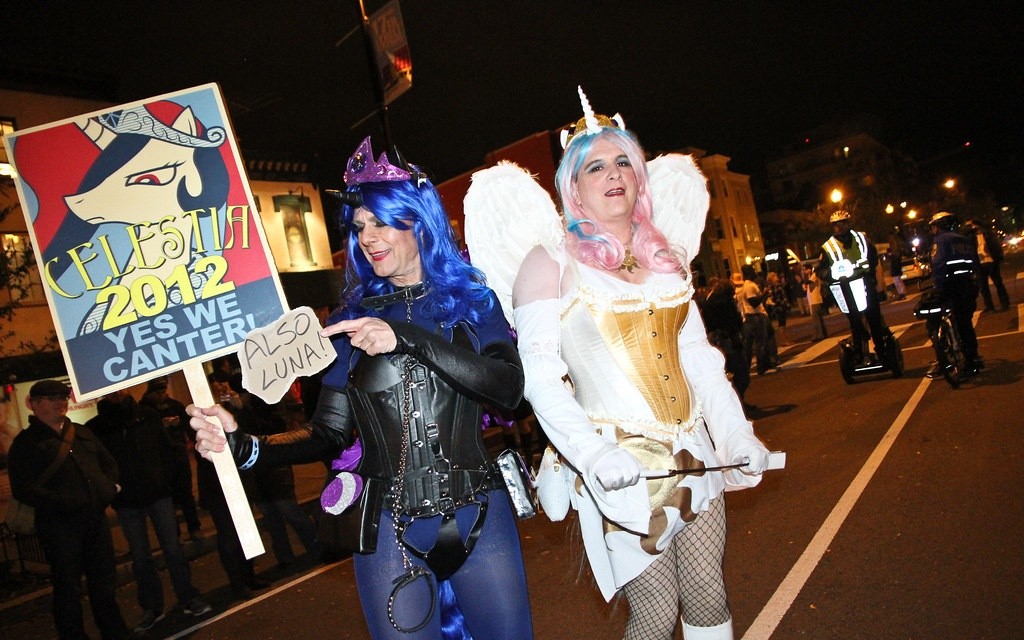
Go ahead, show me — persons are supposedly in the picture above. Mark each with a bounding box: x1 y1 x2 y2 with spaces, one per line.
511 85 768 640
886 248 906 299
696 262 830 409
7 366 322 640
969 218 1009 313
186 136 534 640
926 212 978 377
814 210 889 366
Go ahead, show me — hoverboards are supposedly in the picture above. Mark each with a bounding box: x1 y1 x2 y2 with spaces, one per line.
823 260 904 384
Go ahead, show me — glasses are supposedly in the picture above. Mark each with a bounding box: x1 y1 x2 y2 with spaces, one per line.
34 394 70 402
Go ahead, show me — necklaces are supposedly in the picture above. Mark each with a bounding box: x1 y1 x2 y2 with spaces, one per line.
616 237 640 273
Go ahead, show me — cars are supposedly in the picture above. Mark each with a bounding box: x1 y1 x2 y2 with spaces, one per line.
901 257 931 284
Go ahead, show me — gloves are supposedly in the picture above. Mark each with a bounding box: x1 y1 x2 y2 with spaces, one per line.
592 443 643 492
737 449 769 476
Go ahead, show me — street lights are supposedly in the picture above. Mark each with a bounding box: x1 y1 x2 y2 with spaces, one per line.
825 189 842 229
886 203 903 256
908 210 917 235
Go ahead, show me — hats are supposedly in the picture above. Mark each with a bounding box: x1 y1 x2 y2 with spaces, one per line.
730 272 744 285
30 379 71 397
148 377 167 392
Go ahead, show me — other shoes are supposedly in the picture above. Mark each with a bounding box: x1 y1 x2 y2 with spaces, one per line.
759 368 780 375
862 355 874 367
125 630 147 640
242 577 271 590
812 334 828 342
895 293 907 300
311 544 343 564
983 306 995 312
230 585 249 598
277 552 296 576
963 363 978 378
742 402 762 417
1001 305 1009 311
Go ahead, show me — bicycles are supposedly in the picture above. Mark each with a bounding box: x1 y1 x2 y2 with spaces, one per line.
914 279 984 389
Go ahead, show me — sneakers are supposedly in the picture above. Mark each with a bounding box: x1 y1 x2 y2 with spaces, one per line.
133 608 166 632
181 597 213 615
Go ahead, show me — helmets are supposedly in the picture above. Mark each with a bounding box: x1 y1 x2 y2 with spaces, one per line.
928 211 955 224
828 211 851 224
